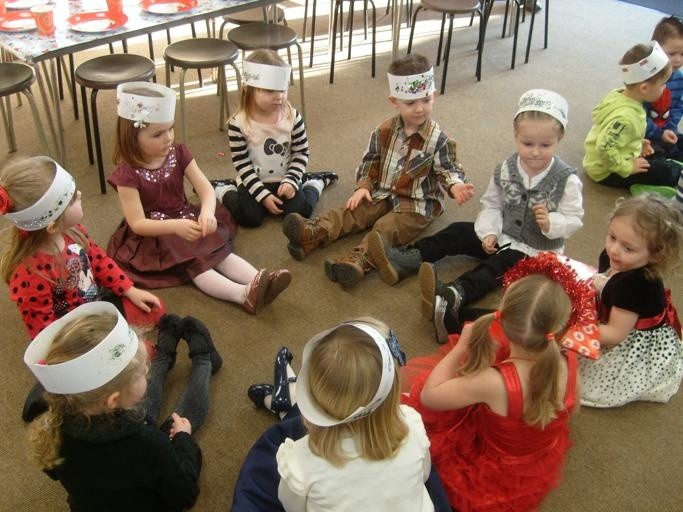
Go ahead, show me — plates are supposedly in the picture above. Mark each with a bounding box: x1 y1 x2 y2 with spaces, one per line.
66 10 128 34
136 1 199 16
0 0 50 34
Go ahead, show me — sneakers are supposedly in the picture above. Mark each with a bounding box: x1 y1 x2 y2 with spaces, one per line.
264 268 291 306
182 317 222 374
152 313 184 369
666 158 683 170
241 269 270 315
629 183 675 201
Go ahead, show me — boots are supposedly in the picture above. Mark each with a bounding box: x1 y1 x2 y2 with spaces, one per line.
325 248 374 286
368 230 422 287
419 261 465 321
283 213 327 261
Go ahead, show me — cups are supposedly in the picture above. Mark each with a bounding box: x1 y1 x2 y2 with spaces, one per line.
104 1 125 16
29 5 56 37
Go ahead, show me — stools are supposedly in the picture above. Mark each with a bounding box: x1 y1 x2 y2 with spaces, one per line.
1 1 306 173
301 1 550 95
75 54 156 195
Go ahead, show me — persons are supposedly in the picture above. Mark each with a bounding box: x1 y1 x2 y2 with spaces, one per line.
643 15 683 144
22 312 223 512
433 193 683 408
366 89 586 321
232 316 453 512
282 53 475 288
0 155 168 423
402 273 583 512
581 39 683 201
192 48 339 228
106 81 292 316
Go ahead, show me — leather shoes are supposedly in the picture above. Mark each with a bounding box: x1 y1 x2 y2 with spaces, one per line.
22 381 48 422
271 348 296 412
248 385 281 416
433 295 449 344
192 179 237 192
303 172 339 189
439 286 462 328
